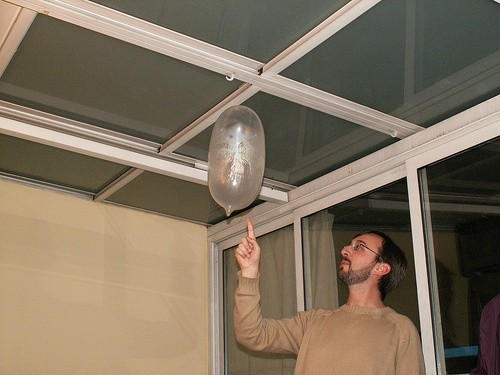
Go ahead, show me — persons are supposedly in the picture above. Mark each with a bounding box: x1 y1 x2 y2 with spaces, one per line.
232 217 426 374
476 272 499 375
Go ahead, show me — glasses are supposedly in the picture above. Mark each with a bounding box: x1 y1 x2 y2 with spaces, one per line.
344 243 382 258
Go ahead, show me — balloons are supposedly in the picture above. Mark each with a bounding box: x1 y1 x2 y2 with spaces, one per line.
206 105 267 216
